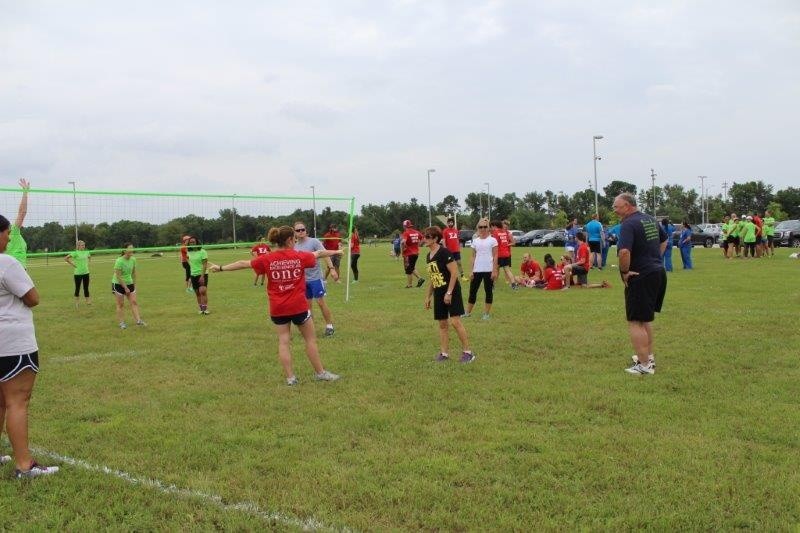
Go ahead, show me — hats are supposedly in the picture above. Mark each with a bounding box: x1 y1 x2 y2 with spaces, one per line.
746 215 753 220
403 220 413 227
741 215 746 219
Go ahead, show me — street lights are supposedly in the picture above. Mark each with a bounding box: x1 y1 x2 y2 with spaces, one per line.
427 169 436 227
650 169 657 221
698 175 708 224
232 194 237 251
68 181 79 251
484 182 490 221
310 185 317 238
593 134 604 214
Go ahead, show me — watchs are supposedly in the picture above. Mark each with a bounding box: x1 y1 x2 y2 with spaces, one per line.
446 292 452 295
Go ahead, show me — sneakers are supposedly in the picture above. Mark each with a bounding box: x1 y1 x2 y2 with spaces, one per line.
624 361 654 376
16 460 60 480
0 455 12 464
631 353 656 368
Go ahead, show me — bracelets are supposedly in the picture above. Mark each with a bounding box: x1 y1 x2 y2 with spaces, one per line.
620 270 630 274
219 265 223 271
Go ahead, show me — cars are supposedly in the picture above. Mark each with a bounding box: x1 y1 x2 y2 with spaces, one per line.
458 230 474 247
772 220 800 248
671 223 722 249
507 228 584 247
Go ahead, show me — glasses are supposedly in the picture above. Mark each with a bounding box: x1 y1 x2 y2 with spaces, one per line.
478 226 487 229
295 228 305 232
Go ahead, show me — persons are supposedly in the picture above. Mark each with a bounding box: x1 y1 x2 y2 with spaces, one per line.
8 177 776 331
423 227 476 365
1 214 61 482
612 193 668 376
209 225 344 384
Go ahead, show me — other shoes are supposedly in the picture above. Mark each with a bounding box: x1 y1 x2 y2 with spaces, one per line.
460 312 471 318
185 287 193 293
481 312 490 320
120 320 126 329
136 319 145 326
560 285 571 291
197 309 211 315
460 351 476 365
315 370 340 381
435 352 449 362
601 279 612 288
287 376 297 385
326 326 334 337
415 278 425 287
